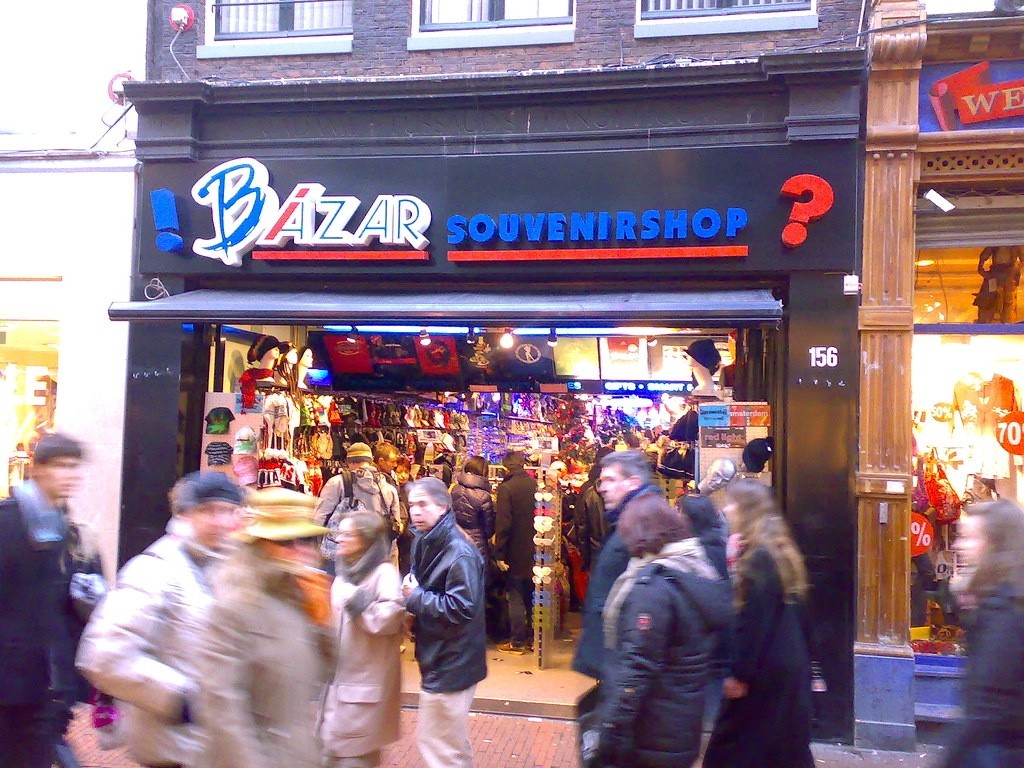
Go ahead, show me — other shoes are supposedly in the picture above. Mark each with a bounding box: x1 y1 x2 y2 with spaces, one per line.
400 646 405 653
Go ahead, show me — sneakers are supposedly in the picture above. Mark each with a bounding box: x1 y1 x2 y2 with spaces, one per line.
526 644 534 651
496 641 524 655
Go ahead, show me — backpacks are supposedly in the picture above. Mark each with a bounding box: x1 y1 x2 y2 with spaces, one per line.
318 471 368 561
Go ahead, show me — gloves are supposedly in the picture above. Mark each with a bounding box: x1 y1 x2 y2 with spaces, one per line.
178 694 202 724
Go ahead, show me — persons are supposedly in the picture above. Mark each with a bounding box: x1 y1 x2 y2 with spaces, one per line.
494 452 538 655
318 440 400 526
315 512 404 768
698 458 736 494
75 471 243 768
973 245 1024 324
401 477 488 768
684 339 720 392
935 500 1024 768
200 487 330 768
248 335 313 388
367 404 470 429
451 456 495 604
374 443 414 498
573 449 816 768
0 435 83 768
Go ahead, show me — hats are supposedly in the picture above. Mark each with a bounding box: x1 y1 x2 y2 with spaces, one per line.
233 456 259 486
252 335 279 362
205 442 233 466
683 349 722 375
345 441 372 459
227 487 332 542
233 425 256 455
205 407 235 434
743 437 774 473
279 341 295 363
263 390 470 487
683 339 716 368
297 345 312 364
657 410 697 478
170 470 242 504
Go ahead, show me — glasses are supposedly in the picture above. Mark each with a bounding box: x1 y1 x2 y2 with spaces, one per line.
531 484 560 629
336 530 360 538
